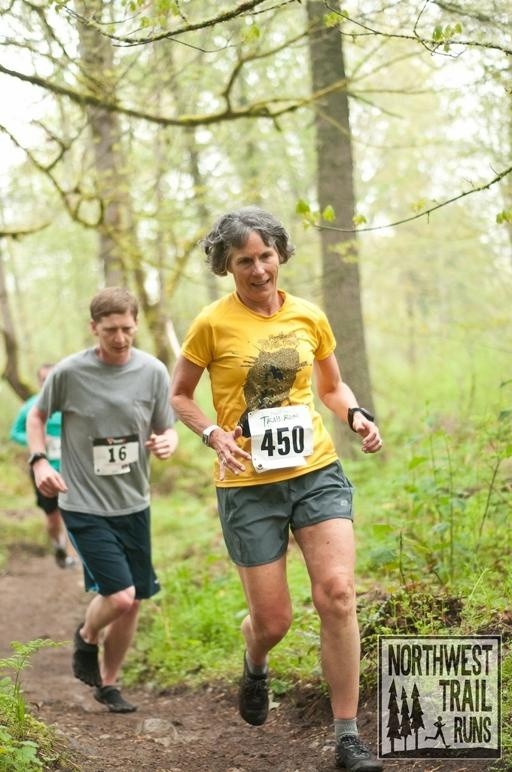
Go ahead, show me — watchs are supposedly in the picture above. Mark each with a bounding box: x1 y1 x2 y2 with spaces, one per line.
346 408 375 434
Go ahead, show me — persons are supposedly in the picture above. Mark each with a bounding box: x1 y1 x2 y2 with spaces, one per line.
166 210 388 771
27 287 177 710
9 362 75 568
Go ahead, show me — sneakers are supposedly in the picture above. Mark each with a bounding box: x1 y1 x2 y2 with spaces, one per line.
52 541 74 566
335 736 382 772
74 624 136 712
239 648 269 726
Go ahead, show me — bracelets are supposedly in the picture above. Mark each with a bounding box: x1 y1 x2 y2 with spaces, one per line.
29 453 50 466
203 423 216 445
